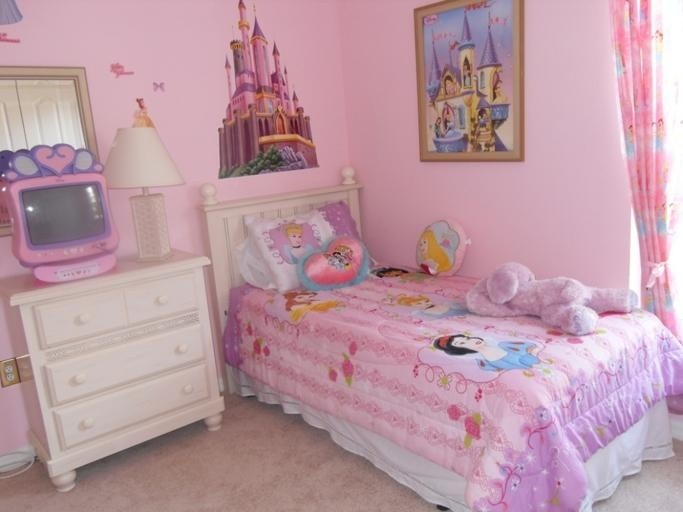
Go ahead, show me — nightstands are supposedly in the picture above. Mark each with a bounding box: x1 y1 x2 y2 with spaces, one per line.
2 245 226 493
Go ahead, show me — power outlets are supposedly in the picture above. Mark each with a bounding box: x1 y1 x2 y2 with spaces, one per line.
0 358 21 387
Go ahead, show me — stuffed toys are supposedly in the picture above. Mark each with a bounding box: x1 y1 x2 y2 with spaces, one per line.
465 261 640 337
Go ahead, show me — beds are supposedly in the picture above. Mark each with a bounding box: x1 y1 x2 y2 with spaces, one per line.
197 165 682 512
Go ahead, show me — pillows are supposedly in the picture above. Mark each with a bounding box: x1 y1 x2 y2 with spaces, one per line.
297 235 370 292
416 218 472 278
235 200 362 294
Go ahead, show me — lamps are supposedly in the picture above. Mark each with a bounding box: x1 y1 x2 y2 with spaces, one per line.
104 126 187 262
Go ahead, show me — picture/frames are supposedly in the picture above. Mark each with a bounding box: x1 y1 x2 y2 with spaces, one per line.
217 3 321 180
413 1 525 162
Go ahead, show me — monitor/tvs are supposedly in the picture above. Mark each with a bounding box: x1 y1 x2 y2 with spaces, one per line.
7 173 120 284
